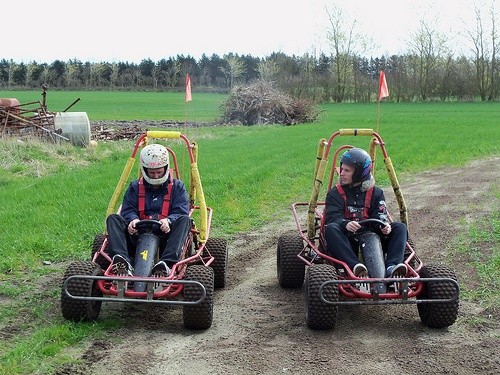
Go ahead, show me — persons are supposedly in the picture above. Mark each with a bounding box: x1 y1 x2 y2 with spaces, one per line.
106 144 192 290
324 148 407 287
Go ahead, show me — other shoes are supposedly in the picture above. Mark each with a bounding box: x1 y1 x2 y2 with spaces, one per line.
386 263 407 284
353 264 369 289
152 261 172 287
113 254 134 285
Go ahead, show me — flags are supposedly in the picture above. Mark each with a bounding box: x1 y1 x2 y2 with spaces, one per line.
185 74 192 102
378 71 389 101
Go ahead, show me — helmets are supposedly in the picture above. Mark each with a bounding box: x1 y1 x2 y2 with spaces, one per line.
140 144 170 185
340 148 372 182
